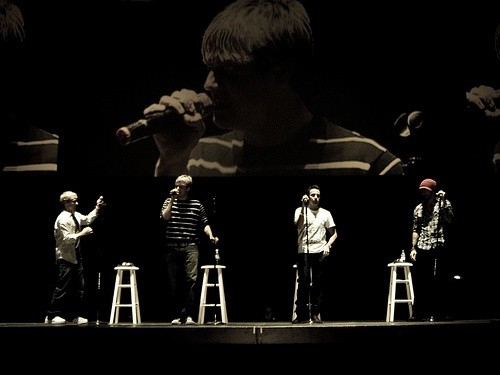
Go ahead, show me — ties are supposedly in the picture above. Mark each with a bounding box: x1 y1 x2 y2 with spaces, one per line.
71 213 80 248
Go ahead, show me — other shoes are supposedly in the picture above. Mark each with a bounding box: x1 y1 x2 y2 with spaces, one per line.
185 316 195 325
311 315 322 323
410 316 421 321
171 318 181 325
292 317 310 324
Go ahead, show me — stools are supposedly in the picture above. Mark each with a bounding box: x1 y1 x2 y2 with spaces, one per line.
198 265 228 325
386 262 414 323
293 265 320 322
107 265 141 325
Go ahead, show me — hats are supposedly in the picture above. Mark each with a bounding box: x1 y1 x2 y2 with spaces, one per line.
418 178 437 191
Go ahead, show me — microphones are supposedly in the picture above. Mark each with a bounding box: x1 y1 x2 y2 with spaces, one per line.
115 93 213 146
170 192 177 195
304 199 308 202
439 194 443 198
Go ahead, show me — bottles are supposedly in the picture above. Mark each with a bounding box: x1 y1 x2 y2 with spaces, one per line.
401 250 406 262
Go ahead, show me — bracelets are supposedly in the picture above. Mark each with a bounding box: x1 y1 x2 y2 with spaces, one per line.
412 247 415 249
301 213 304 216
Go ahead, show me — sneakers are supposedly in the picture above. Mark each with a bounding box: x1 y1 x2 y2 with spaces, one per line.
44 315 66 324
71 316 88 324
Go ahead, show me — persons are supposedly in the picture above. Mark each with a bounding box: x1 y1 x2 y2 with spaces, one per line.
0 0 59 171
464 24 500 169
407 178 453 322
45 191 105 324
291 185 337 323
161 174 219 325
143 0 405 176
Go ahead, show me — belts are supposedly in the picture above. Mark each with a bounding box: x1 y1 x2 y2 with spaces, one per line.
167 242 195 246
